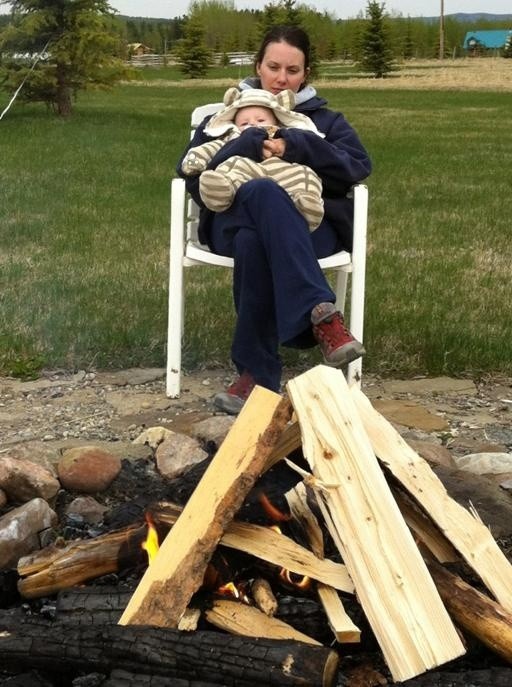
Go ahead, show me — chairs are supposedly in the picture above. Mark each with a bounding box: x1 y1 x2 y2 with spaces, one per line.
165 103 369 400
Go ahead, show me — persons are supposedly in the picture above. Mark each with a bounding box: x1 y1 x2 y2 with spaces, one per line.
175 26 372 416
180 88 326 234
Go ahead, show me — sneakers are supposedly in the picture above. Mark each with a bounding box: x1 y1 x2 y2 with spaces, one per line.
305 302 366 367
214 371 256 413
